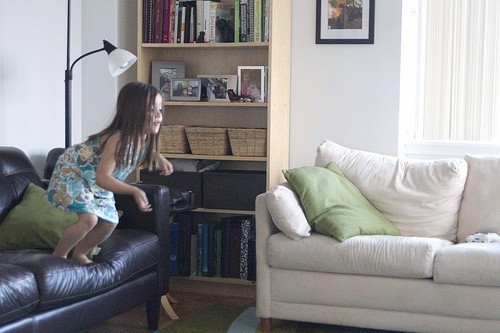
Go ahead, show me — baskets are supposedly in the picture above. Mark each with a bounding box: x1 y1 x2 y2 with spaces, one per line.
183 126 232 156
156 124 191 154
227 128 267 157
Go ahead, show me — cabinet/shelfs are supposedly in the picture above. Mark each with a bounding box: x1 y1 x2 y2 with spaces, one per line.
136 0 291 300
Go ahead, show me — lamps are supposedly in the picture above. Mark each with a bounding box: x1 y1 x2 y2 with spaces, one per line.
64 37 138 150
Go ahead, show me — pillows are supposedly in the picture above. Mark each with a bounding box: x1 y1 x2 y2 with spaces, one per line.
268 181 310 239
279 162 399 241
0 181 123 263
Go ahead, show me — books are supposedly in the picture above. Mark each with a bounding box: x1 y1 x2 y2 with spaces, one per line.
170 217 257 282
143 0 268 43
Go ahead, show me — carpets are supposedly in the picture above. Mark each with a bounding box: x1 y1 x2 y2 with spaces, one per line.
156 298 381 333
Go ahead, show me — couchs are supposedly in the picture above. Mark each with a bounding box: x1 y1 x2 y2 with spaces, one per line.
254 140 500 333
0 144 171 333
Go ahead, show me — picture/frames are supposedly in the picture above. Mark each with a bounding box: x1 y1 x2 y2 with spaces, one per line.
314 0 375 45
237 64 267 102
151 59 186 101
169 78 201 102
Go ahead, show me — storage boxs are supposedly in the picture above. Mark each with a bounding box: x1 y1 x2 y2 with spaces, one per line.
203 170 265 211
139 166 203 207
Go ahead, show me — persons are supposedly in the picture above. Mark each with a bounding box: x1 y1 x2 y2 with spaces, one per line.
44 82 175 264
161 72 262 101
